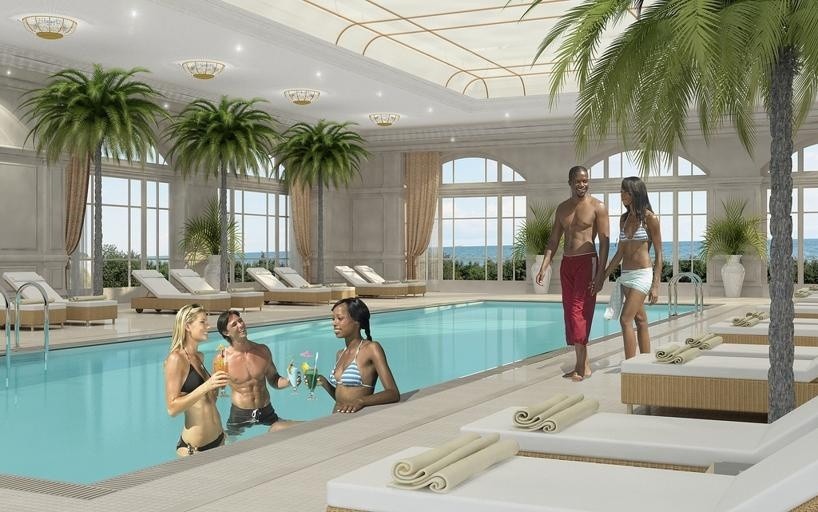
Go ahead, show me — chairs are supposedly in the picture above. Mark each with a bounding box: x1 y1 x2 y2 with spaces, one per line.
3 271 117 326
621 287 814 416
335 265 409 299
458 396 818 476
1 287 67 332
354 265 426 296
273 267 355 301
131 269 231 316
247 267 331 305
325 427 818 510
170 268 265 312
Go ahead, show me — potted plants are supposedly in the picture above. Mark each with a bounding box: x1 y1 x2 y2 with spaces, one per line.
179 196 241 290
696 198 768 300
508 202 565 295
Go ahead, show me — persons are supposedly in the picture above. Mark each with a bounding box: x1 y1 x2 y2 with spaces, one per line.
214 310 301 437
588 175 663 361
536 164 610 382
163 302 230 457
303 296 402 415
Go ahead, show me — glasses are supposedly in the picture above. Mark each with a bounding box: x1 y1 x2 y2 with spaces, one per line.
185 303 200 319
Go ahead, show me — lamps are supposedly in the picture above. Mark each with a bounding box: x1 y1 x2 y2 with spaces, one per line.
21 13 80 41
284 88 320 105
181 57 226 81
369 111 400 127
